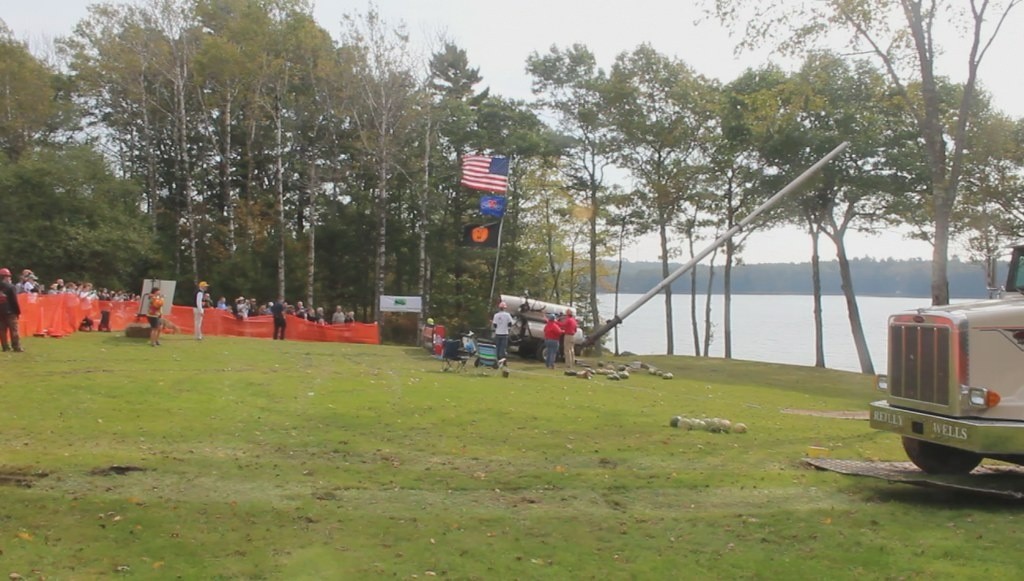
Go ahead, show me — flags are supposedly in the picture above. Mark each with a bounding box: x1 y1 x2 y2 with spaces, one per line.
461 155 509 192
480 196 506 217
465 224 500 247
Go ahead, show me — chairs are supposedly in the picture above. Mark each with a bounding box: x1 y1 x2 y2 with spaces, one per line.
440 334 509 377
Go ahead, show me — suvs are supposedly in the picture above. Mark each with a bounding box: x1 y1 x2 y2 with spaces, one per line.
867 244 1024 475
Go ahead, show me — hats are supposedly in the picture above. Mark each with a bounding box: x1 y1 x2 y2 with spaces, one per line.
199 281 210 286
29 275 38 280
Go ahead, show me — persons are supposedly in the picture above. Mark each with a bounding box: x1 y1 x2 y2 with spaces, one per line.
202 293 272 319
332 306 354 324
271 296 287 340
48 278 141 332
16 269 44 294
543 314 565 369
146 287 164 346
0 268 24 351
283 301 324 324
194 281 210 339
556 310 577 367
492 302 512 366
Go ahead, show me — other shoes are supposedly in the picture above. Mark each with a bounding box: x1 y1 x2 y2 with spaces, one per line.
499 358 506 363
13 348 24 351
156 340 160 345
3 347 11 352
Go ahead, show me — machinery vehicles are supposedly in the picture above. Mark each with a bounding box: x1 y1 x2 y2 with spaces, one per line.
490 140 852 364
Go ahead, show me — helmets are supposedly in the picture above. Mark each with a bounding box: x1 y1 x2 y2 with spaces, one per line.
0 267 11 275
427 318 435 325
566 310 572 316
548 314 556 320
499 302 507 308
